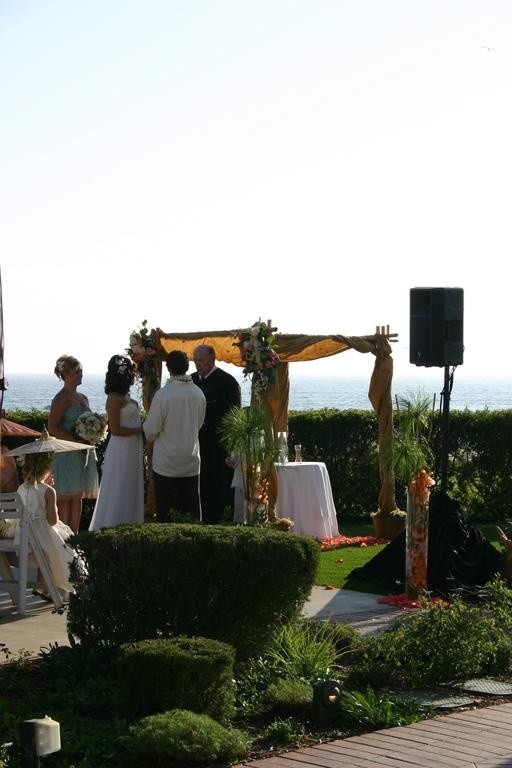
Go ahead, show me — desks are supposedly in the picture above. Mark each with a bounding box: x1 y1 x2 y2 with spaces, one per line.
275 462 341 538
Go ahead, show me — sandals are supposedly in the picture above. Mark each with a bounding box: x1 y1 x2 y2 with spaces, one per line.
32 587 53 602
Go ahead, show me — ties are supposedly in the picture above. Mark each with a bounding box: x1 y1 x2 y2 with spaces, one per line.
198 376 205 391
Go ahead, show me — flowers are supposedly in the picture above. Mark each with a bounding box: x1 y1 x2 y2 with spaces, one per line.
70 411 108 455
231 322 280 408
125 320 159 387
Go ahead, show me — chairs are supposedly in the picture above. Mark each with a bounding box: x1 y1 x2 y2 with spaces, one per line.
0 492 64 616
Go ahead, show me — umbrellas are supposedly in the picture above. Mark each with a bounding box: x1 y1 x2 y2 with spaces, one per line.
5 422 99 459
0 417 41 438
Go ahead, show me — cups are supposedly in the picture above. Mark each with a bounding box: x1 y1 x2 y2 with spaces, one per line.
295 445 302 463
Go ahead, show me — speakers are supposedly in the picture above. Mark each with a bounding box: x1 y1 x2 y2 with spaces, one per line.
409 287 465 367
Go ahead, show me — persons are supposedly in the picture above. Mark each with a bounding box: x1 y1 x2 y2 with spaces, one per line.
185 344 242 528
140 349 208 524
47 353 103 536
87 353 150 533
14 451 89 604
